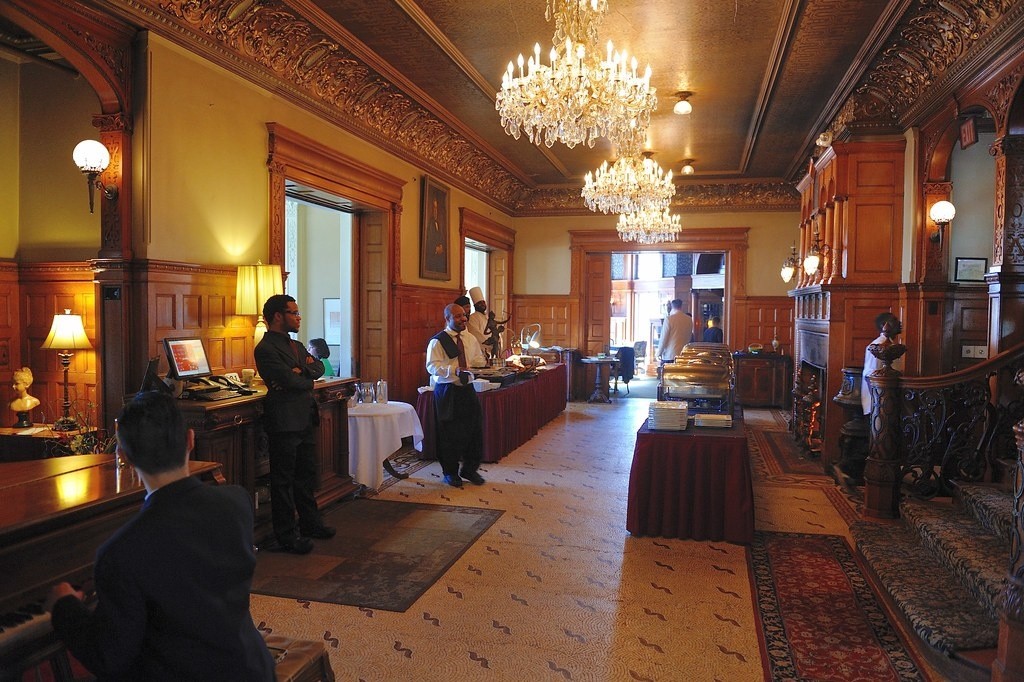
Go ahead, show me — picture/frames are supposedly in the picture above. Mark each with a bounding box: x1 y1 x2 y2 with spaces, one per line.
419 174 451 281
955 257 988 282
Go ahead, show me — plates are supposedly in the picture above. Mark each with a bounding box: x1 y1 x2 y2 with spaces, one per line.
648 400 689 431
694 413 732 428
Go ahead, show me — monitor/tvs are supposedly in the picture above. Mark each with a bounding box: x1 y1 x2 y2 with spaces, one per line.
141 354 161 392
163 338 212 379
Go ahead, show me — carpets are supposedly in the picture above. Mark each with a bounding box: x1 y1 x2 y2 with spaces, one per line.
743 530 932 682
746 425 836 489
248 441 506 612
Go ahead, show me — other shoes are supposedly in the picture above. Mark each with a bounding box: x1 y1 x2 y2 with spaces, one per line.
460 467 485 485
300 525 336 540
283 535 313 555
443 472 462 487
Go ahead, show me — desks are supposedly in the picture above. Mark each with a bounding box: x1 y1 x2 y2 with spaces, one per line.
627 392 755 546
0 423 98 461
417 363 567 463
347 400 425 496
579 358 620 404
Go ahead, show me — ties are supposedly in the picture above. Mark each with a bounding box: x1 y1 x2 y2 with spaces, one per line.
455 334 469 386
288 338 299 360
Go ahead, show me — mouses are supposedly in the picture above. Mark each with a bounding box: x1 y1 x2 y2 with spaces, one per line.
239 388 252 395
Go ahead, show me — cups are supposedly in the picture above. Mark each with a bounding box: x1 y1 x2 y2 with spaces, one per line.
242 369 255 387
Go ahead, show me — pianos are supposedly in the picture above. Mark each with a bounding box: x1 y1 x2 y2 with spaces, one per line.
0 453 228 682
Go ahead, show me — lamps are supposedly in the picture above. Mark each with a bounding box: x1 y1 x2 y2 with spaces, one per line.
73 140 118 214
930 200 956 251
38 309 94 431
520 323 542 348
673 91 692 114
679 159 694 175
581 140 682 244
781 224 831 283
495 0 657 148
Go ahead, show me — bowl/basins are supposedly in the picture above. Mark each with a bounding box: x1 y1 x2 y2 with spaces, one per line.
521 358 539 364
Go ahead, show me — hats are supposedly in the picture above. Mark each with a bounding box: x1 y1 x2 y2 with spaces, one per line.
469 287 484 304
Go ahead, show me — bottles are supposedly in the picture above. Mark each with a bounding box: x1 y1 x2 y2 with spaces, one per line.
376 378 388 404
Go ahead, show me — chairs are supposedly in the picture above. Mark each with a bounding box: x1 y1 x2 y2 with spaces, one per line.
610 341 646 394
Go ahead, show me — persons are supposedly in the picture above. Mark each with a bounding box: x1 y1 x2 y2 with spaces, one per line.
47 391 278 682
832 313 903 488
426 286 493 487
7 367 41 412
656 299 724 365
254 294 338 555
484 311 511 359
307 338 335 376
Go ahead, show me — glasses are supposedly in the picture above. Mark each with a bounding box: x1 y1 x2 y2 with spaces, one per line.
285 310 300 317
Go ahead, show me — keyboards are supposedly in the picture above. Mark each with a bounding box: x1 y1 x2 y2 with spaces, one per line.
197 390 241 401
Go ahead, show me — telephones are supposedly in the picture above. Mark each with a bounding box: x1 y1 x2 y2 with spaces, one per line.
190 377 231 391
209 376 249 388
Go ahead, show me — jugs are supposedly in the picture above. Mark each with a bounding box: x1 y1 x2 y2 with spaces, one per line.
356 382 375 403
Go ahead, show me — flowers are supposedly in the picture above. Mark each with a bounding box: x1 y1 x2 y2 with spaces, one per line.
41 389 117 457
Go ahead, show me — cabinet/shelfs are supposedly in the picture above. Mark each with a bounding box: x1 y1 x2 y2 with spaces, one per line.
527 348 583 401
177 376 361 546
733 354 792 409
